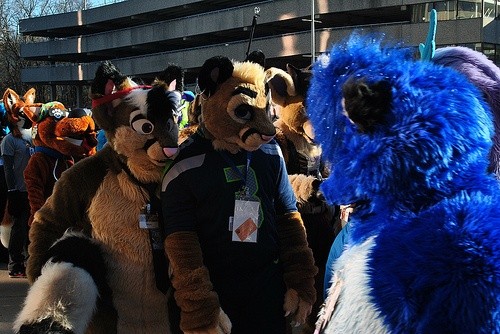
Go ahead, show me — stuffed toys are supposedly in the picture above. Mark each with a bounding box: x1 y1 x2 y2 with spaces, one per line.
10 59 185 333
260 65 346 256
288 7 500 334
13 230 107 333
154 52 318 334
0 87 46 280
20 102 100 263
